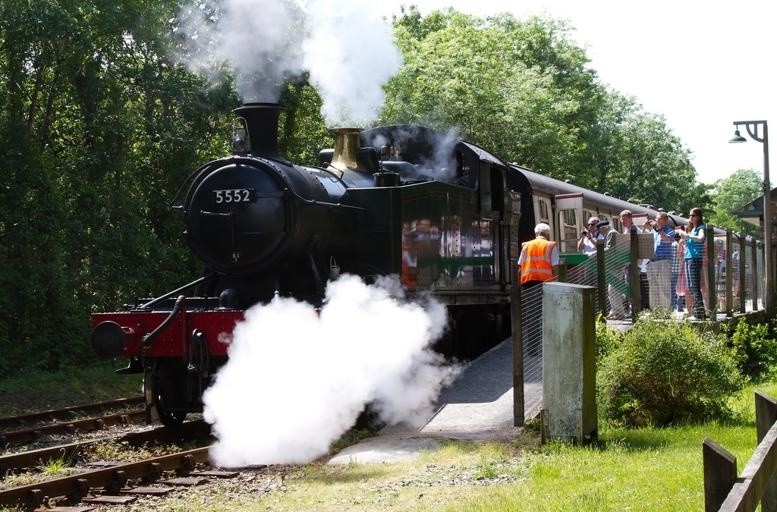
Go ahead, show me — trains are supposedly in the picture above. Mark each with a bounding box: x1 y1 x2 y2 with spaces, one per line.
86 100 771 426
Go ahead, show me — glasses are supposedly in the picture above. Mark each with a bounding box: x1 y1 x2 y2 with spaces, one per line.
689 215 697 217
588 224 594 227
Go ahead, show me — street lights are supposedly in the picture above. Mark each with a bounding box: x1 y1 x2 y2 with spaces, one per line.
727 117 770 311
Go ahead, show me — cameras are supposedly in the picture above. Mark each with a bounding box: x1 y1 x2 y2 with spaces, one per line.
582 229 589 236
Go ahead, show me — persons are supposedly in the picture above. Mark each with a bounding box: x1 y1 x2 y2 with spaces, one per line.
576 207 748 322
401 214 497 291
516 223 565 360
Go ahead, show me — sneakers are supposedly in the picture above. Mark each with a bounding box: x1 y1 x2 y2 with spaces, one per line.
606 315 624 321
677 308 683 311
683 312 713 321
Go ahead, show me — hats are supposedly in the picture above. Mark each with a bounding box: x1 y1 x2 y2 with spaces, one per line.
596 221 609 229
